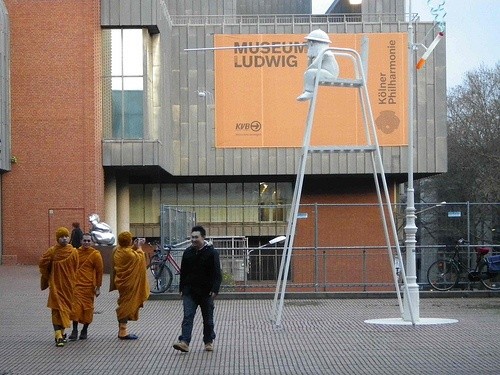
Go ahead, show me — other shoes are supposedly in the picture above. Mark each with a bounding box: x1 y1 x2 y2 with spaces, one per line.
69 330 78 339
79 330 87 340
118 333 137 340
55 333 68 347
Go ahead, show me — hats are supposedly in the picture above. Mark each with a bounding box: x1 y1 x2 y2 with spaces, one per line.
56 227 69 241
118 232 132 247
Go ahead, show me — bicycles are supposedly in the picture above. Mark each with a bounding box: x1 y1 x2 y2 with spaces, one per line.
426 238 500 291
145 244 181 294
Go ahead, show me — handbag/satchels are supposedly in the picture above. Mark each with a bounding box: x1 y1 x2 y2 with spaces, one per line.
41 248 54 290
486 255 500 273
109 249 116 292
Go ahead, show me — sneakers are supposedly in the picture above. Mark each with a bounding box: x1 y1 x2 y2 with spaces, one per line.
173 339 189 352
206 342 212 351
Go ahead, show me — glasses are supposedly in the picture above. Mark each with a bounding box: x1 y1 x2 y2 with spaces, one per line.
83 239 90 243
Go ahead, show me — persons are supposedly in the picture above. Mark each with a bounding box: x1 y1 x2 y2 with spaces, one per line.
89 214 115 245
39 227 79 347
68 222 83 249
109 232 150 339
296 29 339 101
173 225 222 352
68 233 103 340
